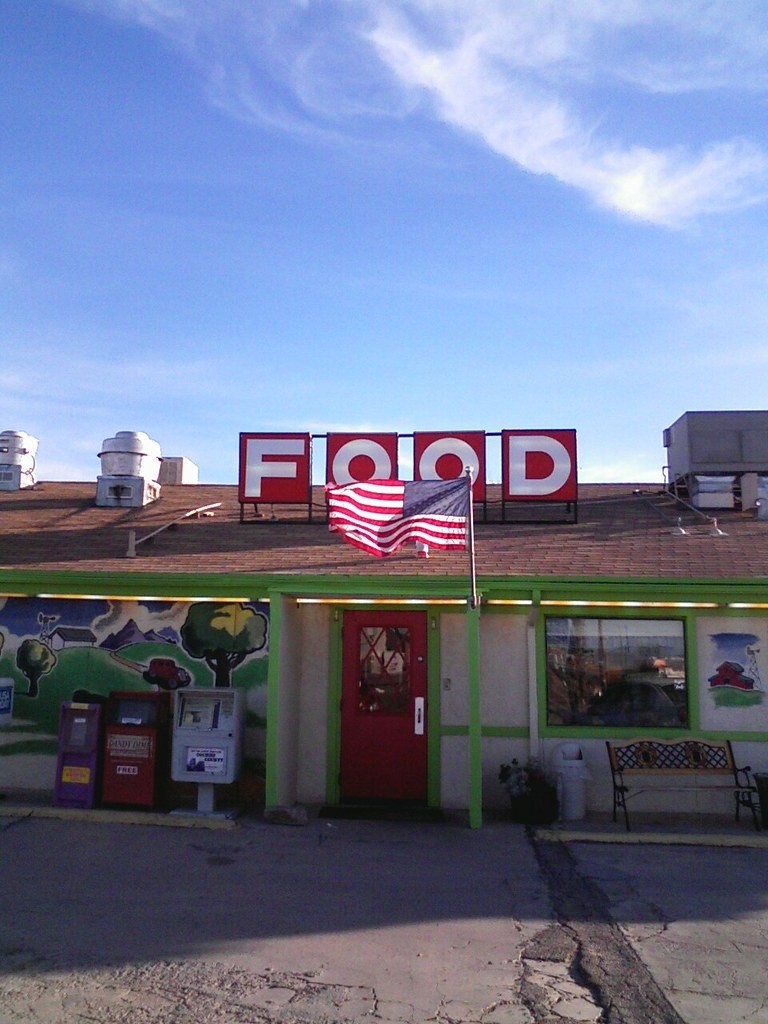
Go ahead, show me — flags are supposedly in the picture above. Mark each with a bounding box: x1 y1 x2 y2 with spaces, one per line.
327 476 470 556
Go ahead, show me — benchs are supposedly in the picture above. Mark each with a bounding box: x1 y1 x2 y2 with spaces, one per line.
605 736 762 831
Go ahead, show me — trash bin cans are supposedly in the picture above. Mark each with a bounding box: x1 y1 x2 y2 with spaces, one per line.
52 686 243 819
556 743 589 821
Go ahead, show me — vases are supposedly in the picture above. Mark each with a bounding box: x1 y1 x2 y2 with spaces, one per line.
508 794 541 824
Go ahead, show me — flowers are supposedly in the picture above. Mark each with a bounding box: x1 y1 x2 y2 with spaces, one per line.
496 759 546 795
